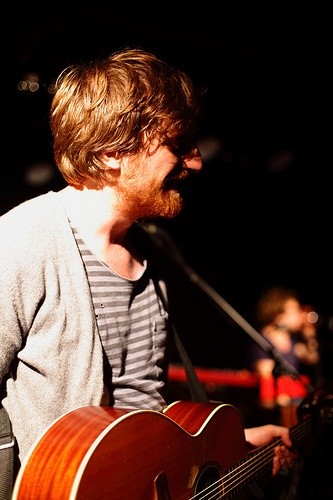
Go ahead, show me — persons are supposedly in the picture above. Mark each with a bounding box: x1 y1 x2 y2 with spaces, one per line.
1 45 300 500
256 287 319 432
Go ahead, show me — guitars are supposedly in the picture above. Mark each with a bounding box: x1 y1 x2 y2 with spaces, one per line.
12 400 332 496
